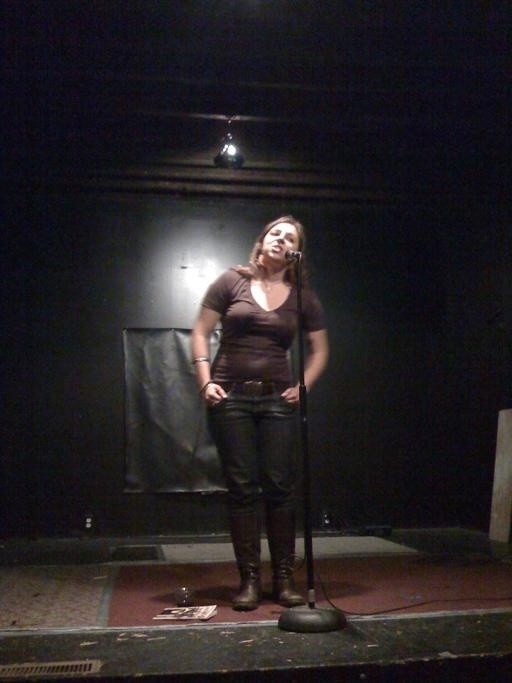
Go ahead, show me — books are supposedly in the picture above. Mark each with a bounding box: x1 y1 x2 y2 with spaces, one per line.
152 603 219 621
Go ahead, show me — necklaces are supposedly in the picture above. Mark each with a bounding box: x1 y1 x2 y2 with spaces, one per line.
262 276 282 293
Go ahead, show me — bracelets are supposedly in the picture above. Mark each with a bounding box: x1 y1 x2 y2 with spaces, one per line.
298 382 310 394
190 356 210 364
198 380 217 399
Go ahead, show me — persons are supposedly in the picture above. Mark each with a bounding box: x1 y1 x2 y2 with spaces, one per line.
189 216 330 611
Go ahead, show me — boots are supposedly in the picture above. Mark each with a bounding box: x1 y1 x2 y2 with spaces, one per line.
268 509 304 607
230 511 262 610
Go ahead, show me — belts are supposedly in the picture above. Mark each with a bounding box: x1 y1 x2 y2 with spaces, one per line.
222 381 291 396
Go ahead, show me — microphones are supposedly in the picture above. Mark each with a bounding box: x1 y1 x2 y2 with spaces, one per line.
285 250 303 259
